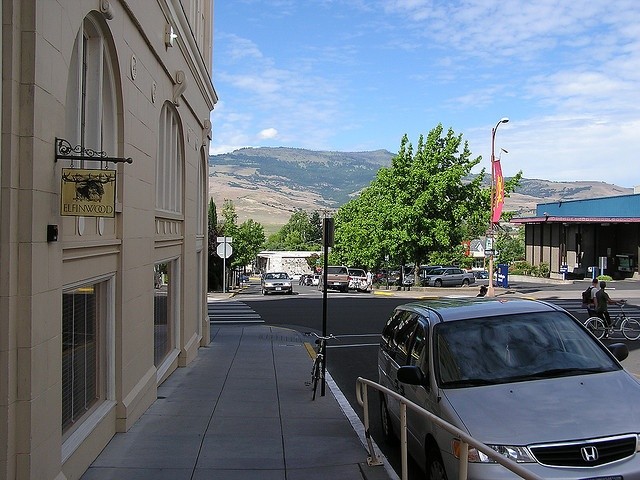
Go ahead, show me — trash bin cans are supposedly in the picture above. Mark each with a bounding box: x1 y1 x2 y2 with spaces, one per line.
592 267 599 279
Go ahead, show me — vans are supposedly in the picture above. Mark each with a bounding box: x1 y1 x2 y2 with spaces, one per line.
418 267 442 275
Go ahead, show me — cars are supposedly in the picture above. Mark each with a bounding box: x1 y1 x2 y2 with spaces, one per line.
424 268 475 287
377 297 640 480
239 274 249 283
348 268 368 291
478 271 488 279
261 272 292 295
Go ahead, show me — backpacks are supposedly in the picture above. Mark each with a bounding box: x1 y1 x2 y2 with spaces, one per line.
582 287 594 307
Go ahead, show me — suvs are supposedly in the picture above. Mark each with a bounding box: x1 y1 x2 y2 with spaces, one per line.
318 266 350 292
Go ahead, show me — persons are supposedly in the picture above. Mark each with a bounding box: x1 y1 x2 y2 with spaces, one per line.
586 279 600 328
594 281 620 338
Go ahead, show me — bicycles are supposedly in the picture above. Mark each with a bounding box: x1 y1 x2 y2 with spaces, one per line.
303 331 342 400
584 299 640 341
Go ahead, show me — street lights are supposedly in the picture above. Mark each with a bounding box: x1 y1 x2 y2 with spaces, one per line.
489 118 509 297
